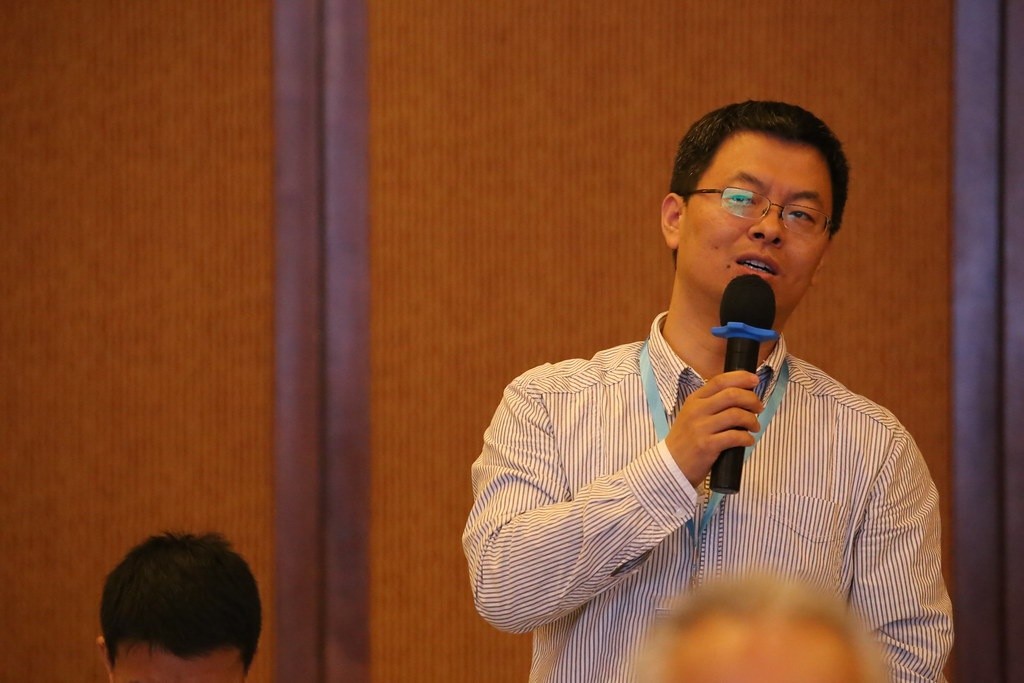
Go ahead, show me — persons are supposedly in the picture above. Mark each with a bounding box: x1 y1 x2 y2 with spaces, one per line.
97 529 263 683
461 99 955 683
624 564 892 683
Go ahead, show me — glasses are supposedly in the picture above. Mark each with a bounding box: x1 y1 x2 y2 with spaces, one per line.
680 187 833 238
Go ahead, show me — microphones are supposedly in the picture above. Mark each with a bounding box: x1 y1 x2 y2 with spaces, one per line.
709 273 777 494
